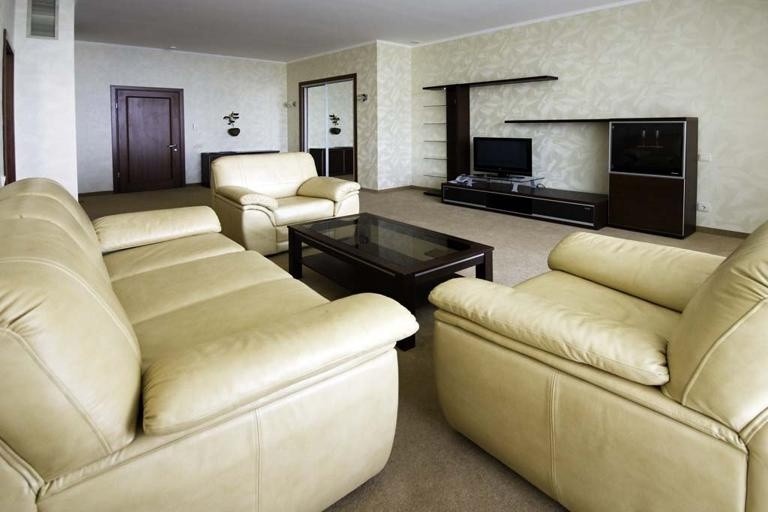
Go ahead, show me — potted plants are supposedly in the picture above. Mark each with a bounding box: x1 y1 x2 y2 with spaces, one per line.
223 111 242 136
328 113 342 135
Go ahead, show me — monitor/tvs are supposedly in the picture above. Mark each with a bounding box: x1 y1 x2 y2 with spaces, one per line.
473 136 532 179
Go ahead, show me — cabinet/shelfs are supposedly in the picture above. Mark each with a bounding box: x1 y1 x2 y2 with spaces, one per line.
422 85 448 197
607 118 698 239
441 179 608 230
460 74 606 124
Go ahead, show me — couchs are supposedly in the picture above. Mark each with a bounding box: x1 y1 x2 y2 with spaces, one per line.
0 178 420 512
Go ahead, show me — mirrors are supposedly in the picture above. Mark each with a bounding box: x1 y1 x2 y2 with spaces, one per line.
298 72 358 182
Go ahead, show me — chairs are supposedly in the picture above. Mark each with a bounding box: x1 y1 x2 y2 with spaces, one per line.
428 220 768 512
210 152 361 257
